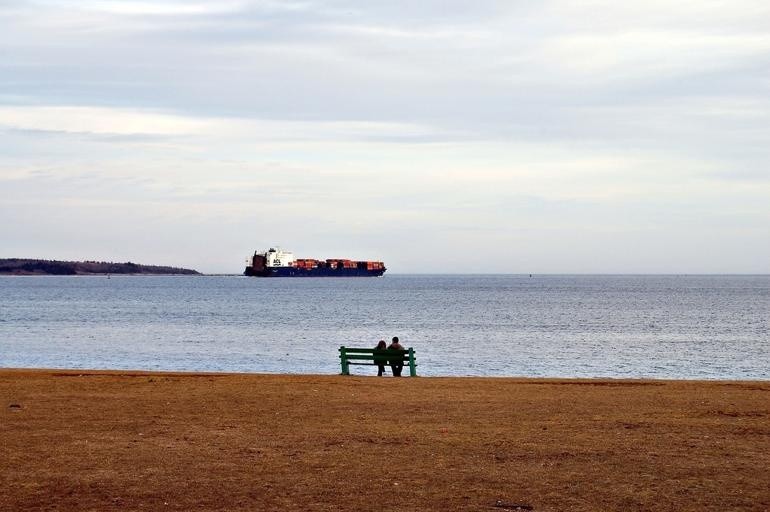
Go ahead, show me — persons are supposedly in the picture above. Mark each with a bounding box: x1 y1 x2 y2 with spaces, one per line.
373 341 387 376
387 337 404 377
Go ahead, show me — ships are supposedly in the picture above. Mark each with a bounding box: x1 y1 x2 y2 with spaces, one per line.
244 244 388 277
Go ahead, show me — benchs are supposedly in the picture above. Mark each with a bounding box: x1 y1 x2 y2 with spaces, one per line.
338 346 417 377
